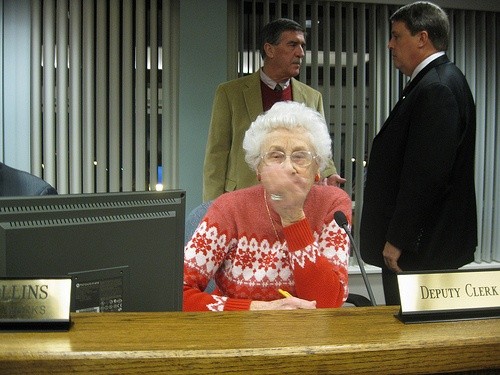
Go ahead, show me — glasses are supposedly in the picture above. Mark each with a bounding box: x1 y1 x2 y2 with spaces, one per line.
259 149 316 166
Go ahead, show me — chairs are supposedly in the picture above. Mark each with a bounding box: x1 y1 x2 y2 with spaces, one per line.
185 200 373 307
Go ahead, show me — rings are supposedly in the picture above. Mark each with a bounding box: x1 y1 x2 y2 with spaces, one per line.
270 192 282 200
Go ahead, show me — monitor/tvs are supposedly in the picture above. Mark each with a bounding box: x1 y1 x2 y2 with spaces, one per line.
0 189 186 311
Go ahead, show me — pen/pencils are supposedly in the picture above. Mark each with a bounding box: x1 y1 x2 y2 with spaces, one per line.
279 289 293 297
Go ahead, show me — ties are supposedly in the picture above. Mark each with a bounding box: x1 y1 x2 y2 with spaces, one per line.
274 82 282 101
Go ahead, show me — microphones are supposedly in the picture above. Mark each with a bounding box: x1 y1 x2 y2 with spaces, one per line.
333 210 377 306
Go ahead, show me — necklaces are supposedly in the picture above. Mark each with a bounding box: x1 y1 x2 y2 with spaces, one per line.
263 189 287 260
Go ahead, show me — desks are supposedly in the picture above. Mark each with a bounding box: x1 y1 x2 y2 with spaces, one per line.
0 305 500 375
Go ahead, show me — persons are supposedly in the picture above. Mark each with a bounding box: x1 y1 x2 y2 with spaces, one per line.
0 162 58 196
202 17 348 202
359 1 479 306
183 101 353 312
